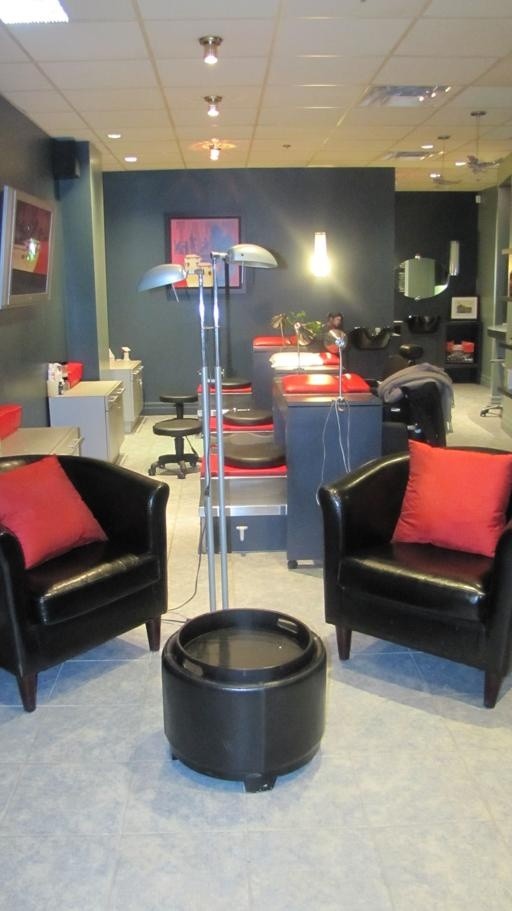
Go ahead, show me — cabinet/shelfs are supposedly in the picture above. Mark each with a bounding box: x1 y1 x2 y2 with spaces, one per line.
0 425 82 457
99 358 144 432
50 381 125 465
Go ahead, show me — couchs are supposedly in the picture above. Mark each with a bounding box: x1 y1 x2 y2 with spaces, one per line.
317 445 512 707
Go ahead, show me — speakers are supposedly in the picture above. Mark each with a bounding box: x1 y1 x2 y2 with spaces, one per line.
48 139 82 181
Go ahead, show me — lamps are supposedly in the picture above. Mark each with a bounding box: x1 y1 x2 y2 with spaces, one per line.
204 95 223 117
308 232 334 280
200 37 221 63
209 139 220 161
271 313 349 402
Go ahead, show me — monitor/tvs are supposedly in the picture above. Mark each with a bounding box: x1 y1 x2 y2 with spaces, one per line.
448 240 477 277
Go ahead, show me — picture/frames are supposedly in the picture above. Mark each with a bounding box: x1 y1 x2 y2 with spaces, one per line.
450 296 479 319
165 211 248 295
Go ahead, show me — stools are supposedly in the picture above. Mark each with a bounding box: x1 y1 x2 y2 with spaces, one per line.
148 392 202 479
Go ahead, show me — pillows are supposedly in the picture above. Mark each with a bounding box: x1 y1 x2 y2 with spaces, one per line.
1 455 106 568
390 440 511 558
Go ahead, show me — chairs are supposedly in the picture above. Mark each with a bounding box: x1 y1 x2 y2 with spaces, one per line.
365 344 423 393
0 456 170 712
